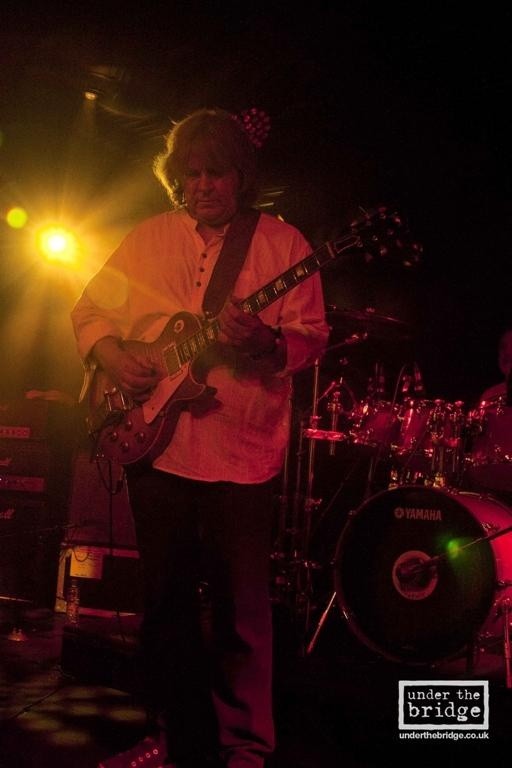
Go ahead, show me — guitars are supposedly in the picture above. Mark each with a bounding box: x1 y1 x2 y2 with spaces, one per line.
84 203 423 466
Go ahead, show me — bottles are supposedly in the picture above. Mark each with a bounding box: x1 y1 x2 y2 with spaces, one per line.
65 579 80 626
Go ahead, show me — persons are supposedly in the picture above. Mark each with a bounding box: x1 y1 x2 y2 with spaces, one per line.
69 109 332 768
455 333 510 505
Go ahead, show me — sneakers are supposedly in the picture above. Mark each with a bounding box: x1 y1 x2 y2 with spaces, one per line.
99 732 166 768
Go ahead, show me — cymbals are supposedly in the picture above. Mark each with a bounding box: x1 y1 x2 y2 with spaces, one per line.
327 307 414 341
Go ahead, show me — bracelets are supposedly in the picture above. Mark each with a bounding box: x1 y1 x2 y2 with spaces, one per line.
253 325 283 361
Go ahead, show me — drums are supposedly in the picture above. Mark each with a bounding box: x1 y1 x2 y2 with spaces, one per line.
395 398 467 480
467 402 510 492
348 395 402 462
333 484 511 669
